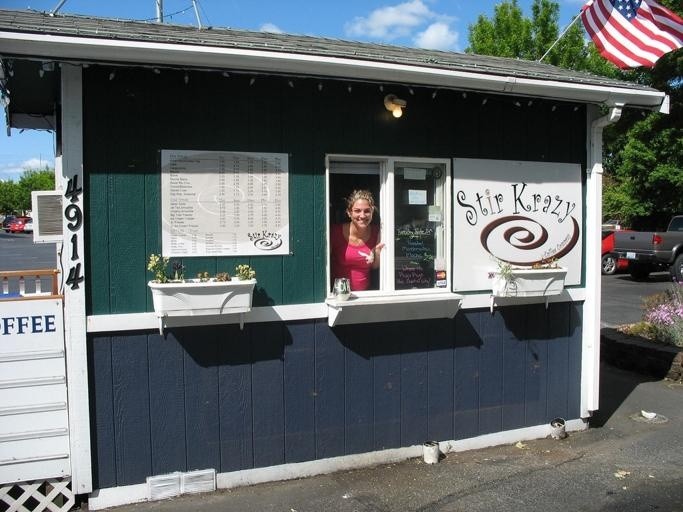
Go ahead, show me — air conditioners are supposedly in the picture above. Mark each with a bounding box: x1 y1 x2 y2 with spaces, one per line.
31 190 65 243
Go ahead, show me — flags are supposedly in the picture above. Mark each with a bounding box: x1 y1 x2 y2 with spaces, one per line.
580 0 683 72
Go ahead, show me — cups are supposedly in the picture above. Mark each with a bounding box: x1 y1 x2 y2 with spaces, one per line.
333 278 352 303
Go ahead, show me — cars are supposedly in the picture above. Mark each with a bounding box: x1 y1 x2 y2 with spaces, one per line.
0 215 33 234
600 219 642 277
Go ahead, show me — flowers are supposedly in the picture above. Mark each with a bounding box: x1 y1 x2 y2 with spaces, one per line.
147 254 255 282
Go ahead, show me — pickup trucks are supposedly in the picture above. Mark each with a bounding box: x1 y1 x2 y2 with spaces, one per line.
612 215 683 282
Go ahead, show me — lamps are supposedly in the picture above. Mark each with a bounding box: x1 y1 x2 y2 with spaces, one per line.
384 95 408 118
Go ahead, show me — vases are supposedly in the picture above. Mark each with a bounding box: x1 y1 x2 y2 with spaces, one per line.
148 277 258 336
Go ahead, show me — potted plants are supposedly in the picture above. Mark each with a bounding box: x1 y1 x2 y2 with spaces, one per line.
484 249 568 315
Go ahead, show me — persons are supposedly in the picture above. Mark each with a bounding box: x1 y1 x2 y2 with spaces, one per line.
328 187 385 291
